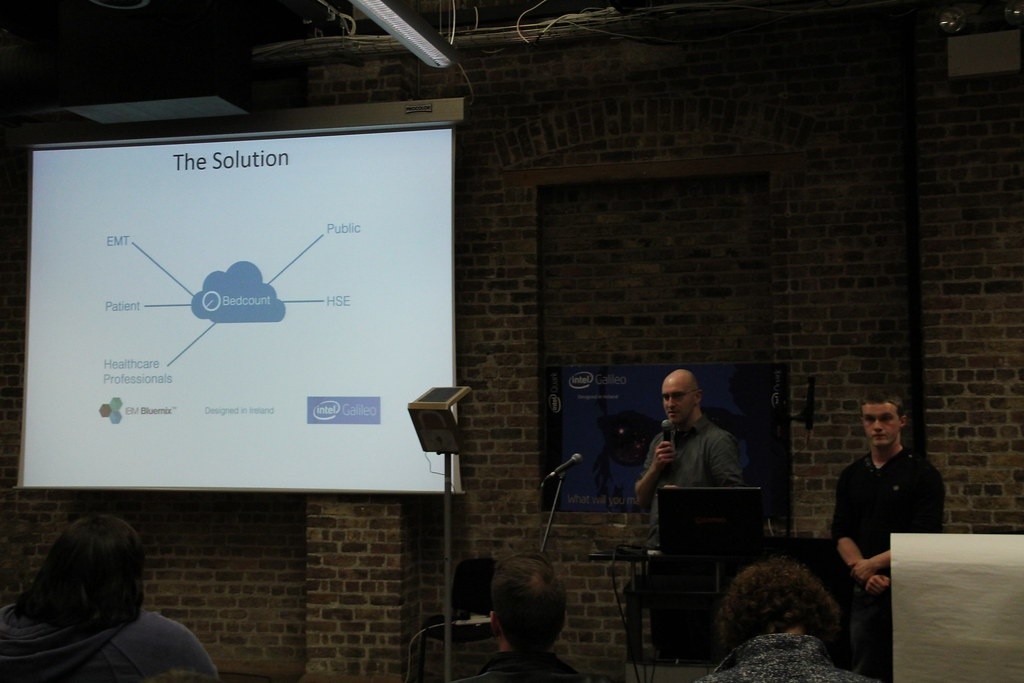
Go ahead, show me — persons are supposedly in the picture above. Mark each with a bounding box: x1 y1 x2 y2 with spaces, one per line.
697 555 883 683
636 369 744 549
0 515 225 683
831 393 946 683
454 553 579 683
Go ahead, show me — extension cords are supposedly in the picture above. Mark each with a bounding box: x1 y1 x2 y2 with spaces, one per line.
454 617 491 625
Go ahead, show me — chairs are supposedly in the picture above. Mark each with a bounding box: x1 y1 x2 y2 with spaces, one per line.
417 557 495 682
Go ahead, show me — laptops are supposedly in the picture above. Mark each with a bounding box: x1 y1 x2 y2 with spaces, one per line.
656 487 764 555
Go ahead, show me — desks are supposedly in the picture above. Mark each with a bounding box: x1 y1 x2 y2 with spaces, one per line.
585 538 850 683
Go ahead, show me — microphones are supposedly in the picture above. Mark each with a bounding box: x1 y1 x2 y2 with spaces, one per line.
661 419 673 471
804 374 817 439
546 452 583 479
